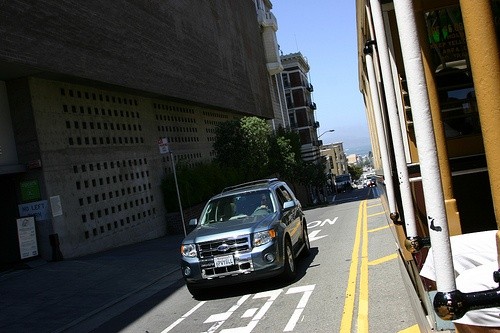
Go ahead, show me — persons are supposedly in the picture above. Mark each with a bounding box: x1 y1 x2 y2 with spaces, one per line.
258 193 273 213
223 201 245 222
344 180 351 193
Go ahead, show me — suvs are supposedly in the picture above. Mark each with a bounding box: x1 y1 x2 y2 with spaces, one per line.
181 177 311 297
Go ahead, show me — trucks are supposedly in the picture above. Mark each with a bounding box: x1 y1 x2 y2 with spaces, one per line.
336 173 352 192
